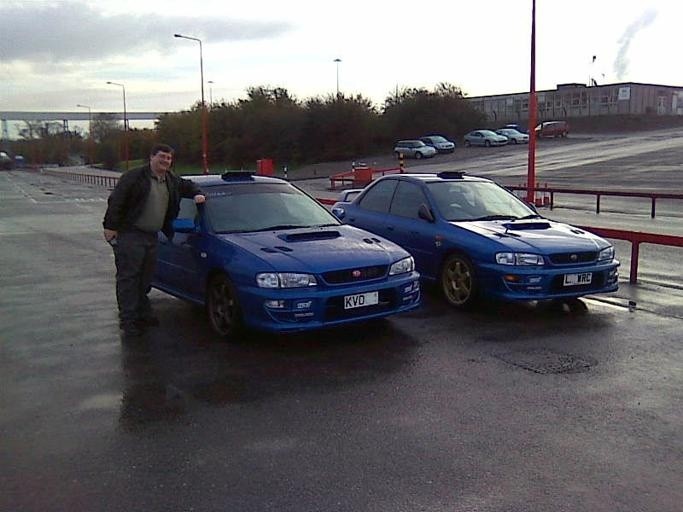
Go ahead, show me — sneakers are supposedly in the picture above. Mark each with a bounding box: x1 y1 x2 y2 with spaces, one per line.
117 315 160 337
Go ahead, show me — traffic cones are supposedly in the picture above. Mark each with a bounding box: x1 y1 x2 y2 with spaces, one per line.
543 182 549 204
534 182 543 207
521 182 527 202
516 182 521 199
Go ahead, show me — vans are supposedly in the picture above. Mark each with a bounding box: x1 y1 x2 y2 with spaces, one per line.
534 120 569 138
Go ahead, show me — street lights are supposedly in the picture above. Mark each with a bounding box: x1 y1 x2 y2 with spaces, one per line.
105 81 128 171
587 55 596 120
333 58 342 97
174 34 209 175
76 104 91 135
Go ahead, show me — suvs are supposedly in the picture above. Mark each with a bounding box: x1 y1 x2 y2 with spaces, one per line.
393 140 436 159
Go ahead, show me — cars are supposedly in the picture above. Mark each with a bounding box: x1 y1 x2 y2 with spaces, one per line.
332 170 621 310
495 128 529 145
147 171 421 343
463 130 508 148
502 122 530 134
419 136 455 152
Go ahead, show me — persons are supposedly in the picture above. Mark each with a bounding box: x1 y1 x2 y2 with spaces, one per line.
102 144 207 336
